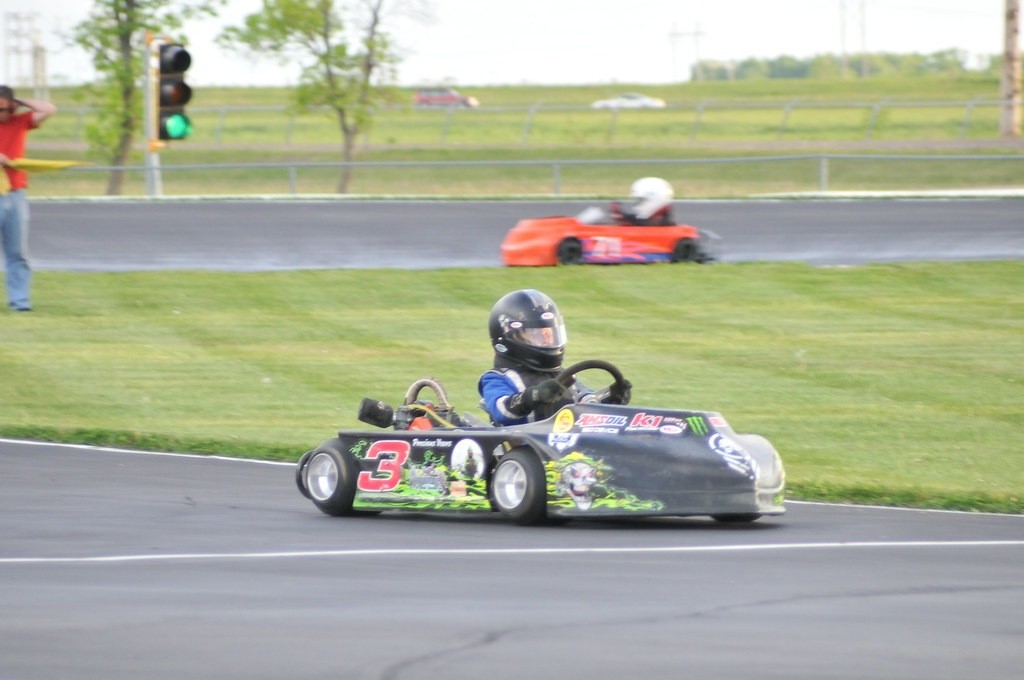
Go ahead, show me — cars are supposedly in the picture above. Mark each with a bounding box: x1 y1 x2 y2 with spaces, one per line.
416 86 479 109
592 92 666 108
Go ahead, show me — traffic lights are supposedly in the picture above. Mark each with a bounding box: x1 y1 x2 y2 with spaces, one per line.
159 43 193 141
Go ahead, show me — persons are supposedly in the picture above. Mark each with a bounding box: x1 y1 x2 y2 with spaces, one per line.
609 177 676 226
0 85 56 311
478 289 632 426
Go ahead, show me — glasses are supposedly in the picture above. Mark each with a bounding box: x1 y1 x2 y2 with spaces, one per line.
0 107 16 113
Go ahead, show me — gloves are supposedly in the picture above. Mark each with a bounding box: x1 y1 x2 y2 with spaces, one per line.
525 379 566 409
602 380 632 405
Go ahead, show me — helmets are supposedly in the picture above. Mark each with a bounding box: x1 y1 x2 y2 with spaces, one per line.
632 177 675 219
489 292 564 373
